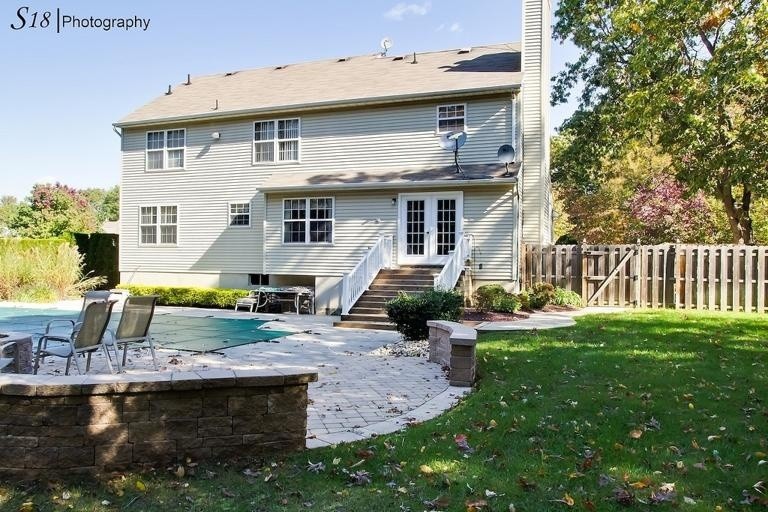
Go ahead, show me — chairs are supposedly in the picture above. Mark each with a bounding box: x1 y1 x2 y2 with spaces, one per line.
32 288 162 376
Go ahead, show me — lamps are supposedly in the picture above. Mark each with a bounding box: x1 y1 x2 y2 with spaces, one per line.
212 131 221 140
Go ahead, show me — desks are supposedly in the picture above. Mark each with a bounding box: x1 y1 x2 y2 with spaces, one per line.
249 286 315 316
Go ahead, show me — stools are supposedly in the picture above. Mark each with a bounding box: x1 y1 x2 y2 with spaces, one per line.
235 299 257 314
